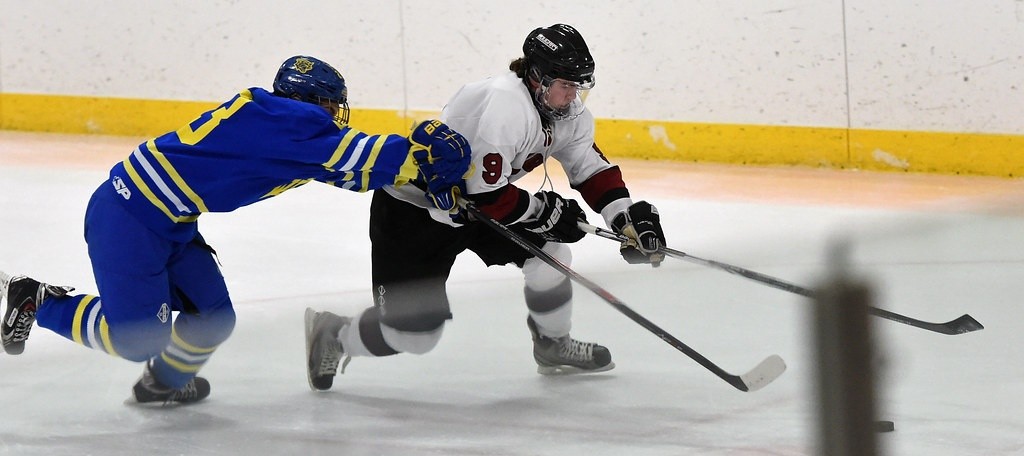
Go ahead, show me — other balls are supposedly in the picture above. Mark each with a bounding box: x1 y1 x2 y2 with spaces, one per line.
871 421 894 433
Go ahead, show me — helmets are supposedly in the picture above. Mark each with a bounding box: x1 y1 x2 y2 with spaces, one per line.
273 56 351 128
523 24 595 124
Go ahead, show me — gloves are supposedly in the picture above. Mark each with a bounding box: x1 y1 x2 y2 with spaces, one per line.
522 190 589 243
407 118 475 212
611 200 666 264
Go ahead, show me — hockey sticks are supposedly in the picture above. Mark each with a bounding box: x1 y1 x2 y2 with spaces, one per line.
576 221 985 335
456 195 787 393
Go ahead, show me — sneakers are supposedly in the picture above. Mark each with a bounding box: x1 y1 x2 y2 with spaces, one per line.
304 306 353 393
528 314 617 376
124 358 210 410
1 271 76 355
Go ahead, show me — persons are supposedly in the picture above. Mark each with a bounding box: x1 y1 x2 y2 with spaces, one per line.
304 24 646 394
0 56 476 403
